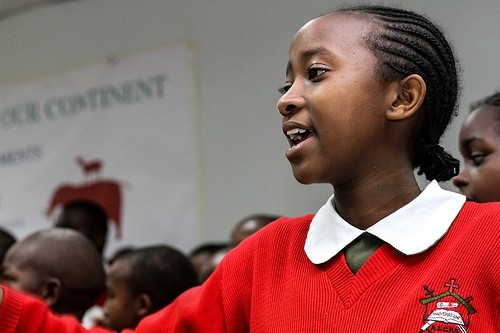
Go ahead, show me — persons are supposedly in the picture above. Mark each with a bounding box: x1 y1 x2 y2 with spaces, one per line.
0 92 500 333
0 6 500 333
